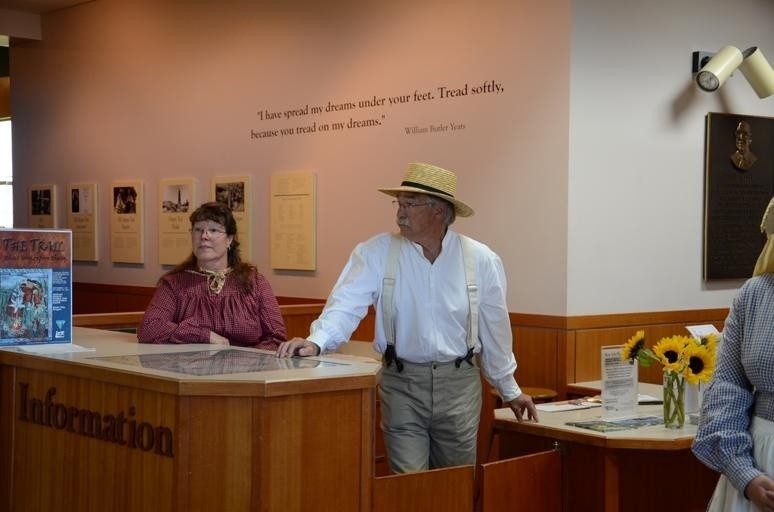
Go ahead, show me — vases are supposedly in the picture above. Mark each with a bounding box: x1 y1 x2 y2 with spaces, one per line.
661 373 686 429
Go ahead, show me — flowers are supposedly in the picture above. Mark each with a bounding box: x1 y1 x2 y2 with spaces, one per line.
618 326 719 429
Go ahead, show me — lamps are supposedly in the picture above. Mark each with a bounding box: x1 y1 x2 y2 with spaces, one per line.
690 45 774 99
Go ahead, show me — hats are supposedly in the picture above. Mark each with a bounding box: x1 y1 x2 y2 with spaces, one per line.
377 161 475 218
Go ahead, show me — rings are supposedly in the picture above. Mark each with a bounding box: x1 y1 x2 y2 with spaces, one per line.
303 339 308 348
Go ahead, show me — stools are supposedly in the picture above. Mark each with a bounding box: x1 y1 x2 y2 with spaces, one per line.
473 387 559 512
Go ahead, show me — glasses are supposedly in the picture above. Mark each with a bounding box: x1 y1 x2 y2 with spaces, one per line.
392 200 433 212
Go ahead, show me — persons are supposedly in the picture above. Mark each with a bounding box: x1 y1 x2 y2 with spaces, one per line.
274 161 540 478
730 119 759 170
690 197 774 512
232 190 242 211
137 201 286 352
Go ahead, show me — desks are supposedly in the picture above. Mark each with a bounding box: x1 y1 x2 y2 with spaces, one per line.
482 396 722 512
0 326 475 512
